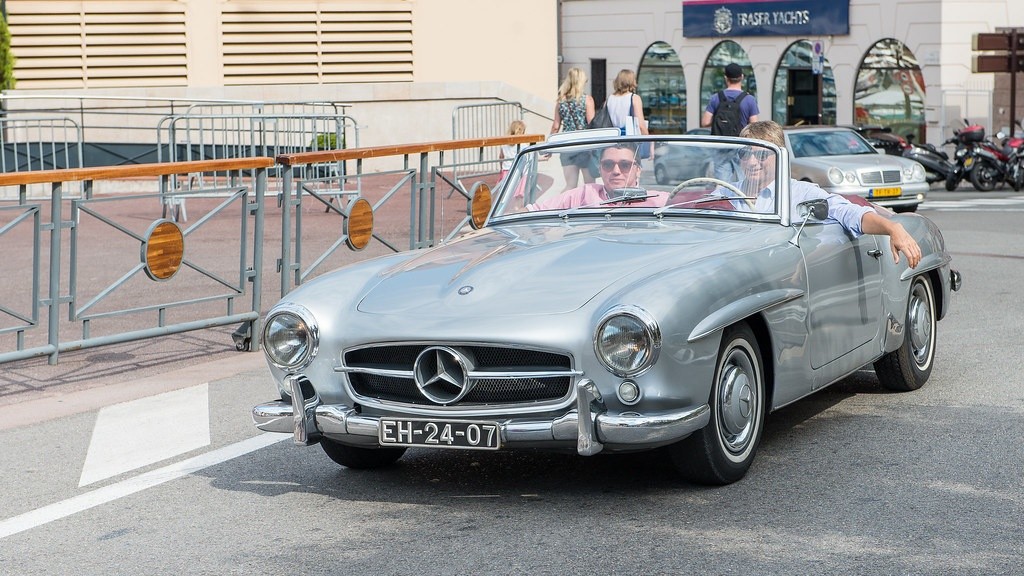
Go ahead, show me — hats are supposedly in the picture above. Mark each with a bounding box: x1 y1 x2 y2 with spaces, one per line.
725 63 741 78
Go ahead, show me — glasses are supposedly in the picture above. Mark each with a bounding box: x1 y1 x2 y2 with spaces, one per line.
737 149 776 161
600 159 636 173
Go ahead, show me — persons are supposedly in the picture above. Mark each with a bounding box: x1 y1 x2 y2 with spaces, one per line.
499 63 921 270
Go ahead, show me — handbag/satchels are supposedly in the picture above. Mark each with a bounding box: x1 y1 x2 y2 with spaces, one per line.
625 92 650 135
587 96 613 128
588 156 600 178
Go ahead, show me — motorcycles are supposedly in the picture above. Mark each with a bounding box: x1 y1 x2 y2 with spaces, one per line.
901 118 1024 192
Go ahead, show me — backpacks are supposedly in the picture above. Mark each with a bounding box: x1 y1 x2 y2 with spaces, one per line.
711 90 749 136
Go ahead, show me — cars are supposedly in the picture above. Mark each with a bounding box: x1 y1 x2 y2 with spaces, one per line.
834 126 907 156
780 124 930 215
653 128 716 186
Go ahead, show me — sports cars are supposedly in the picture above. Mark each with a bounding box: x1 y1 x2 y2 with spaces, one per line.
252 132 962 485
